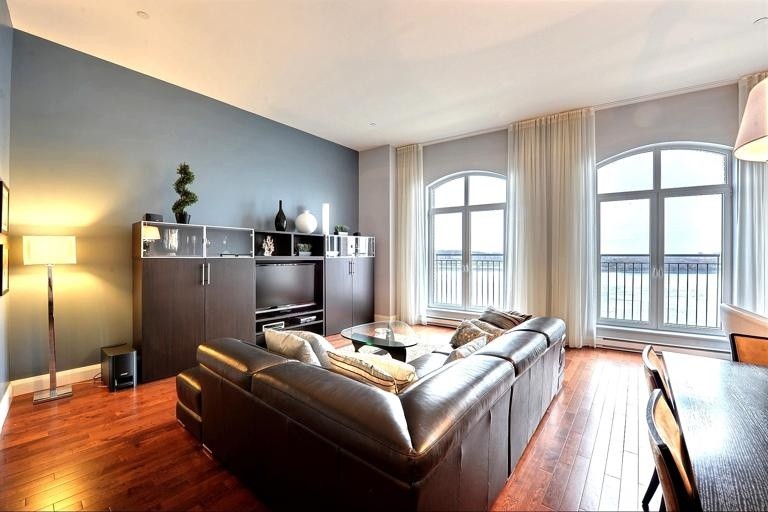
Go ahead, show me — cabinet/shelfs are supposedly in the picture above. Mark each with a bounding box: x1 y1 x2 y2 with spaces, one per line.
254 230 326 348
132 221 255 383
323 236 376 337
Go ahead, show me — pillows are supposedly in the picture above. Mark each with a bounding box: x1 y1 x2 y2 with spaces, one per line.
509 309 528 320
479 307 521 333
448 319 493 347
261 328 321 368
346 351 420 392
473 321 505 341
325 351 399 399
281 327 336 372
444 335 489 365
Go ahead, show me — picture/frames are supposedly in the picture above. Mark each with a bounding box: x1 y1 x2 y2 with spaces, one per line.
0 243 11 298
0 179 10 236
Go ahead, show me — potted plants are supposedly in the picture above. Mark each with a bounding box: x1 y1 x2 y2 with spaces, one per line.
334 223 349 236
170 163 197 224
296 243 312 257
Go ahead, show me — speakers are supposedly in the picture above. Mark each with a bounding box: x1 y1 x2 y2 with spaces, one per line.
101 343 137 393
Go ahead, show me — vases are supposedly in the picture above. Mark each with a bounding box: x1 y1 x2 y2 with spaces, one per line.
274 198 287 231
294 209 317 234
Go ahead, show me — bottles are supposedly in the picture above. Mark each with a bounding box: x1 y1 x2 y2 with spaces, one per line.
275 200 287 231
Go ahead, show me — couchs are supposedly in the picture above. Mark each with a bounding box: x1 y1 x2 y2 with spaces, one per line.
174 316 565 512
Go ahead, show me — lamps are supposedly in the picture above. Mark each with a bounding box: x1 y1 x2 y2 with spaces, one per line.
732 76 768 163
23 234 77 406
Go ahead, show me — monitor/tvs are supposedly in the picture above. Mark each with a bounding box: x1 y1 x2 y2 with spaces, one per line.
255 263 315 310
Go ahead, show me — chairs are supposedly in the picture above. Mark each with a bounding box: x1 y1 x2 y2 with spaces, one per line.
727 334 768 368
719 303 768 338
645 387 702 512
639 344 675 512
